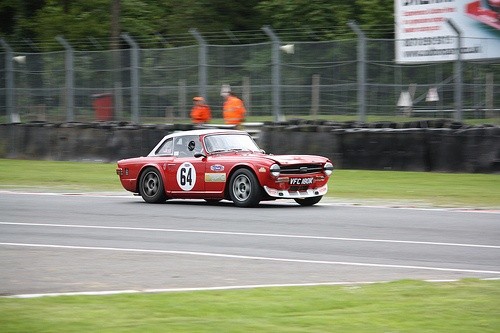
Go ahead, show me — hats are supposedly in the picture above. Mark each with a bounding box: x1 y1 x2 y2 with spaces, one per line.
192 97 202 101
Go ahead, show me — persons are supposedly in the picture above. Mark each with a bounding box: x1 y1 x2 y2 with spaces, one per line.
189 96 211 124
220 84 246 126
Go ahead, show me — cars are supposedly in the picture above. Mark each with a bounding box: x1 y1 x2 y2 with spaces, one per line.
119 129 334 212
